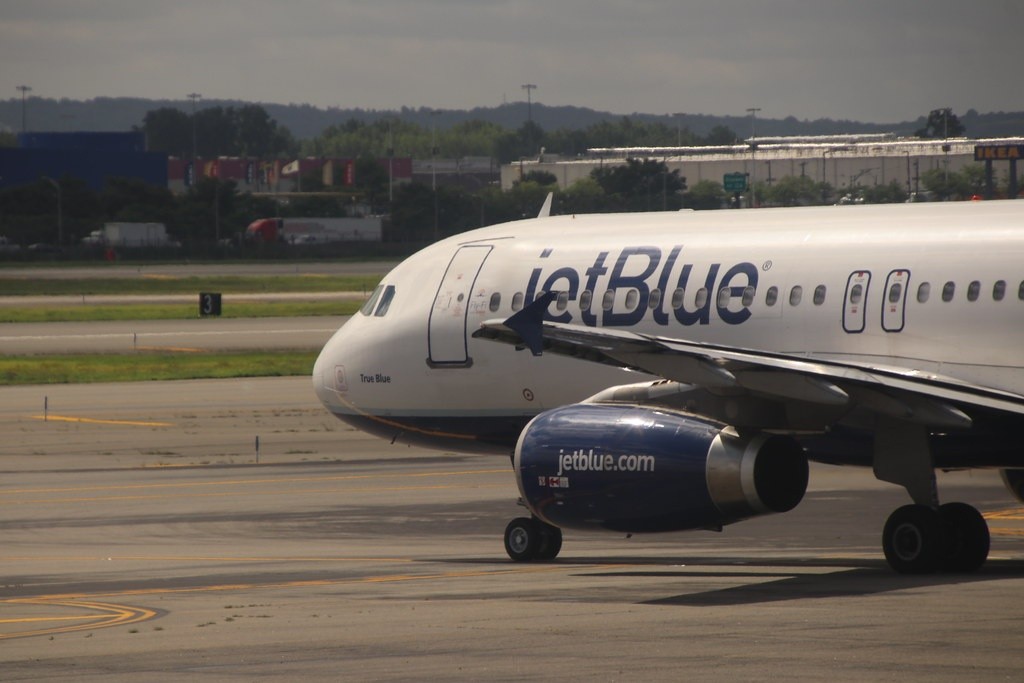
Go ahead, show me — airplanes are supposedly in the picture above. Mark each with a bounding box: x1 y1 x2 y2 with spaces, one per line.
311 195 1023 578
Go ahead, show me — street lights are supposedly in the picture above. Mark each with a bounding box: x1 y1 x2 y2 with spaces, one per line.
16 86 31 132
746 107 762 207
872 146 885 187
186 92 205 167
903 150 911 200
521 84 537 158
821 148 837 184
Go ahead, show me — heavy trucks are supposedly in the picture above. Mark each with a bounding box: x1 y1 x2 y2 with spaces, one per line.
245 214 385 261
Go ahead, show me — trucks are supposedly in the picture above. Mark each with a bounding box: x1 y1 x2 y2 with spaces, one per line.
81 221 171 251
